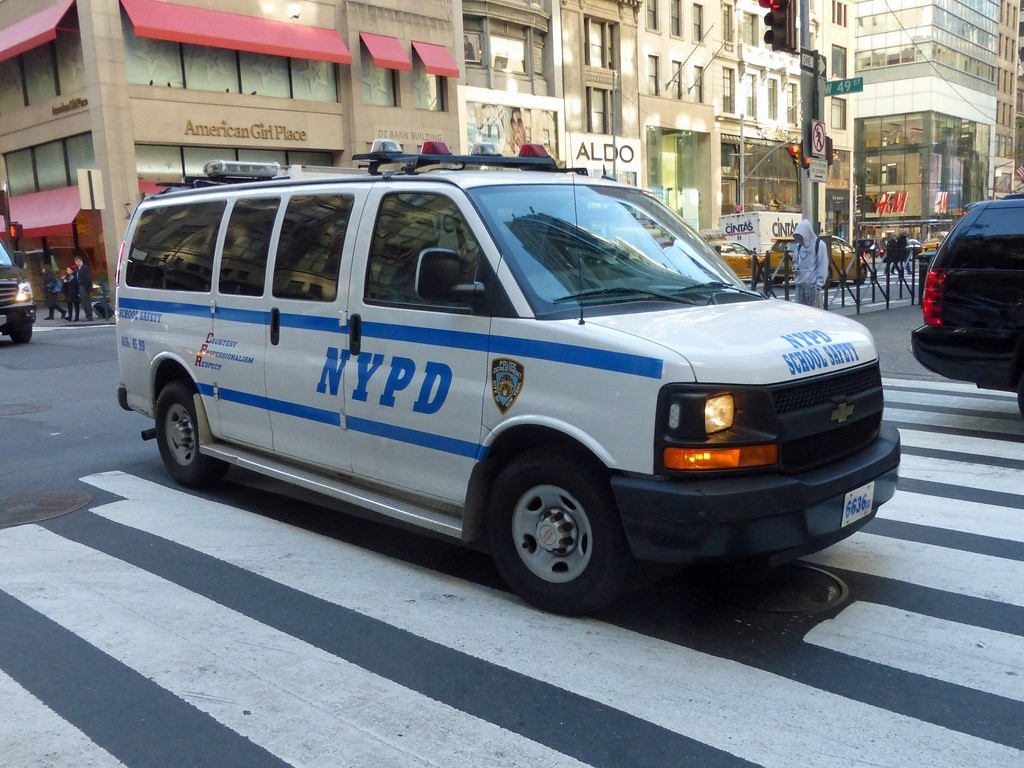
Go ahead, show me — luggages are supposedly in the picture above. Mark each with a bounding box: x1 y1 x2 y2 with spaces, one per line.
89 297 115 320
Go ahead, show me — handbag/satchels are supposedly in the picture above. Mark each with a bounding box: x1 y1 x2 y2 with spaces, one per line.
61 284 68 293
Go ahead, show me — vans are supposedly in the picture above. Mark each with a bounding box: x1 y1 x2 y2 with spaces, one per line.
113 135 905 620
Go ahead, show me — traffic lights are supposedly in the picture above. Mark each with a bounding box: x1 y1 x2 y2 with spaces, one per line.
759 0 796 52
789 145 800 164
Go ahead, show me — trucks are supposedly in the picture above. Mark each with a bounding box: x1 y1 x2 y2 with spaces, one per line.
718 212 802 254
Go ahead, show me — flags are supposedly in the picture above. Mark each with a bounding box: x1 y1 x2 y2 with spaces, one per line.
1016 161 1024 185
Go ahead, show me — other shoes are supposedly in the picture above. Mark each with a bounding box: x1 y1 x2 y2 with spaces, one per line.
84 318 92 321
44 317 54 320
61 310 67 319
73 318 79 322
64 317 72 321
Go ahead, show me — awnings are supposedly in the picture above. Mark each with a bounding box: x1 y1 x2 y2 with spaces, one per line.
359 32 413 71
411 40 460 78
120 0 352 64
0 0 79 63
0 185 82 240
138 181 180 194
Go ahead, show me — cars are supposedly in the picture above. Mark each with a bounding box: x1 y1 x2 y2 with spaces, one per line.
906 239 923 261
760 232 867 289
704 238 766 283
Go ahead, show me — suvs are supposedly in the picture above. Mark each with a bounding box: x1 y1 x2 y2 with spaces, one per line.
907 191 1024 421
0 239 37 344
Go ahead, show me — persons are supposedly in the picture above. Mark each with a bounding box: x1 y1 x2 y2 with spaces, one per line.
42 264 67 320
792 220 829 310
74 256 93 322
464 35 475 60
62 266 80 321
883 230 915 275
